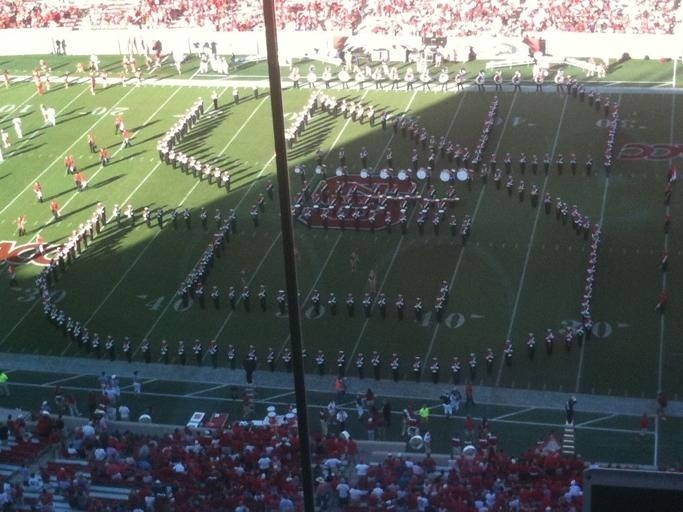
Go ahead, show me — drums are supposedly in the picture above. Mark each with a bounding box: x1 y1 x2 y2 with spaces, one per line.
457 168 468 182
417 168 427 180
397 169 407 180
360 169 368 178
440 169 451 182
295 167 300 173
335 167 344 177
380 169 389 179
315 166 321 175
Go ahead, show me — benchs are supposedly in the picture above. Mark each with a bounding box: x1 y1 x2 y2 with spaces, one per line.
0 432 135 512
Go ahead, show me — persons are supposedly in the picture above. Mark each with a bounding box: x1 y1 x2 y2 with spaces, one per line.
1 1 682 512
